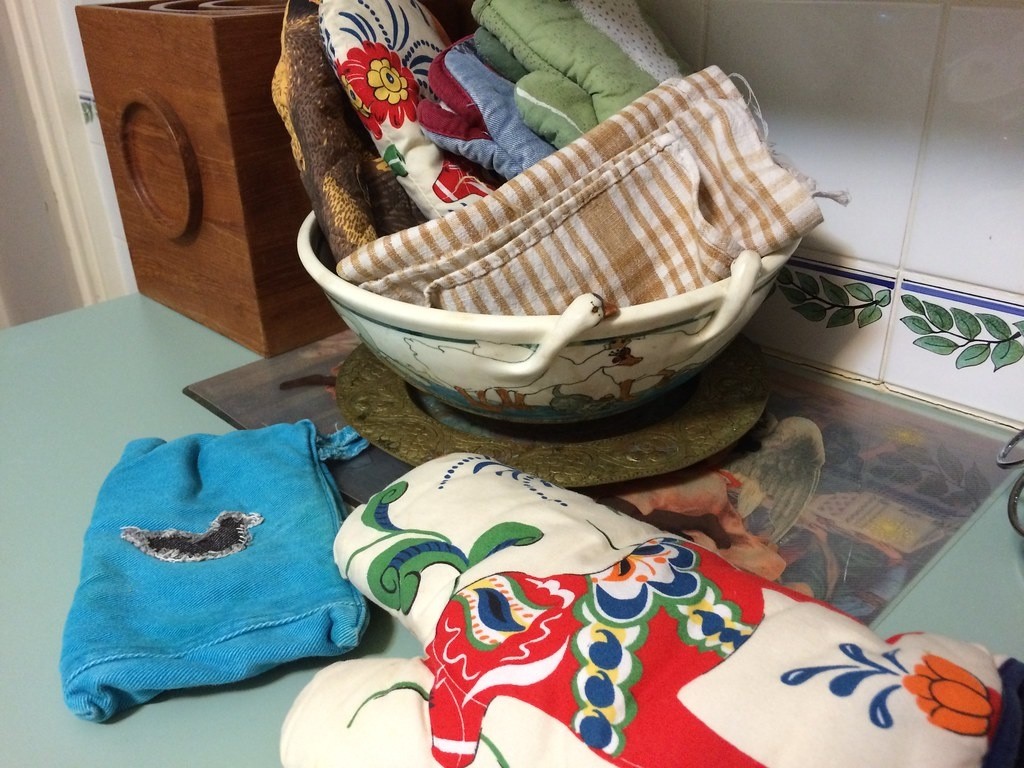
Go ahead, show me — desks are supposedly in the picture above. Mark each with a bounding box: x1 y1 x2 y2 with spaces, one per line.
1 292 1024 768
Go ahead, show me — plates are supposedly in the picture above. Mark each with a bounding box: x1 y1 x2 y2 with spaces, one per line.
335 340 765 489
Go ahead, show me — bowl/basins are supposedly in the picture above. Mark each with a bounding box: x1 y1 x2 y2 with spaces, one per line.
297 210 805 424
